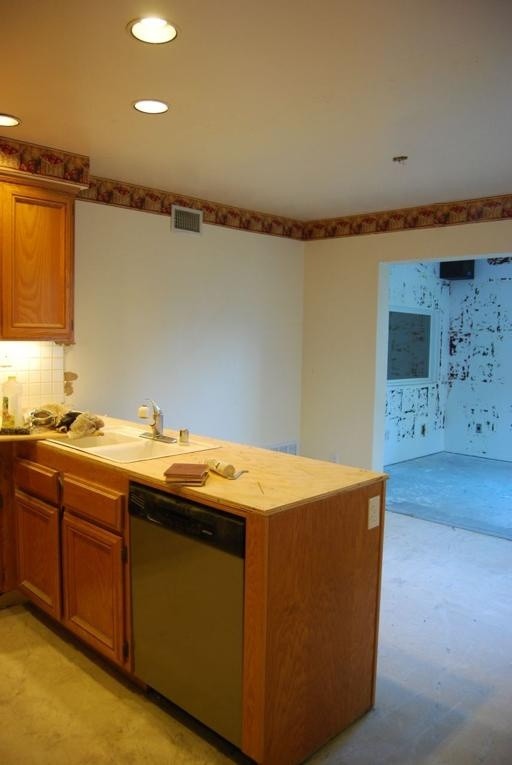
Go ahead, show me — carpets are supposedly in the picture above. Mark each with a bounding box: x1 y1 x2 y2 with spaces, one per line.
382 451 511 540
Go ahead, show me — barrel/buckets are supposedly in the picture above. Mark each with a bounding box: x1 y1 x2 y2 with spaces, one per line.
0 375 24 434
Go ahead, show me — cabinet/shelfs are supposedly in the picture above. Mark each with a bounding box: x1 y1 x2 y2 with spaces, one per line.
0 442 16 609
16 442 131 678
0 173 80 345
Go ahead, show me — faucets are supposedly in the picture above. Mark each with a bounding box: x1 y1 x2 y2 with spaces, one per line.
137 400 164 439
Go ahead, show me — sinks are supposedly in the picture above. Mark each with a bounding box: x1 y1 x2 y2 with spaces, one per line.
85 438 225 465
45 426 149 448
